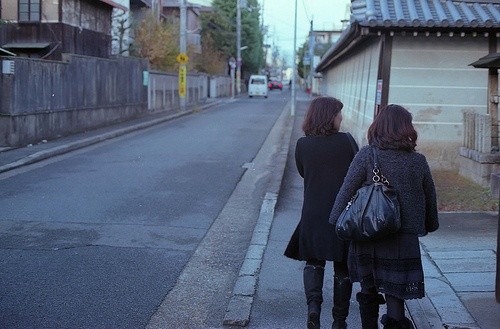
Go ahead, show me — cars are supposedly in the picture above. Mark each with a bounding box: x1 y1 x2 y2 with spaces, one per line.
268 78 283 91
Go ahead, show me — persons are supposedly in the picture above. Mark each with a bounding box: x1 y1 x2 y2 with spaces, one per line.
331 104 440 329
294 98 361 329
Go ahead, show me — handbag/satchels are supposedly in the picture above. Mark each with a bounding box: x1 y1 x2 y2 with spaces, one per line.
336 149 400 243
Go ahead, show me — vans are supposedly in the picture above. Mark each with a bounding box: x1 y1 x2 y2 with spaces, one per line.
247 74 269 98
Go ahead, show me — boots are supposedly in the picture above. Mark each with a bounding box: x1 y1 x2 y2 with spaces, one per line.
303 265 325 329
356 292 385 328
381 314 412 328
331 275 353 329
